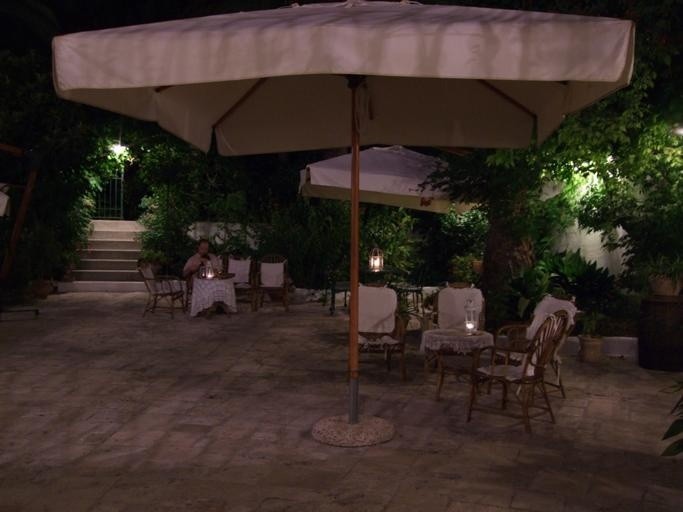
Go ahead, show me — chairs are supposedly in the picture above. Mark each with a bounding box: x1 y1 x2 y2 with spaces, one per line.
321 255 429 317
138 252 290 318
347 279 580 434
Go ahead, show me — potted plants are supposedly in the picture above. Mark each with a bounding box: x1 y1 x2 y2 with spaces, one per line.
577 264 614 366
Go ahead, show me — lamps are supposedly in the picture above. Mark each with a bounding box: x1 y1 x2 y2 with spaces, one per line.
369 248 386 274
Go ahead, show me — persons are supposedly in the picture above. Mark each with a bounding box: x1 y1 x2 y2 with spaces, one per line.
180 238 220 320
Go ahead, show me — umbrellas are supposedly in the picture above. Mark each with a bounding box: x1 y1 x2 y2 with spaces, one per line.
297 142 488 220
46 0 639 424
